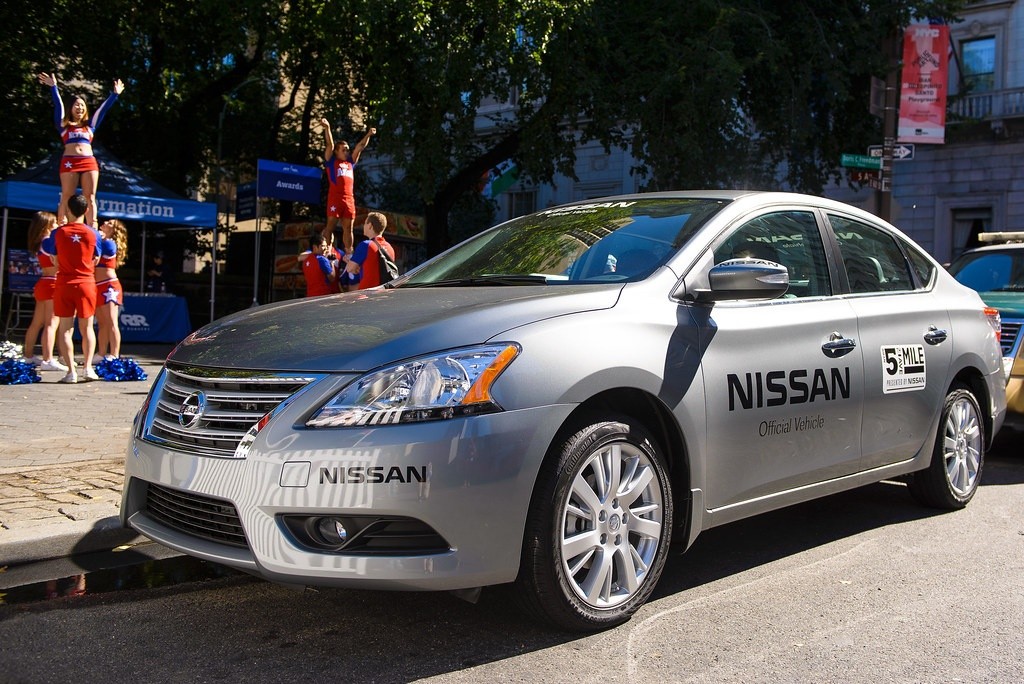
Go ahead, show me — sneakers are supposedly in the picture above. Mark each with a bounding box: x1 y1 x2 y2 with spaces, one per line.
59 371 77 384
83 368 98 379
23 356 42 364
91 354 115 365
39 359 69 372
58 356 77 367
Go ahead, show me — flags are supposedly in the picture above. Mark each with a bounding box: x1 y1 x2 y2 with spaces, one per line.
258 160 321 204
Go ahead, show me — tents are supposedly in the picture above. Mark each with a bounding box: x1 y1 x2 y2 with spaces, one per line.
0 142 218 324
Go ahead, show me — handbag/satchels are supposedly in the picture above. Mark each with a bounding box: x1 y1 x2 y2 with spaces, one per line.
377 248 399 286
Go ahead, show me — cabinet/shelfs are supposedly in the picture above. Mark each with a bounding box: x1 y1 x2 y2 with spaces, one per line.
5 292 43 348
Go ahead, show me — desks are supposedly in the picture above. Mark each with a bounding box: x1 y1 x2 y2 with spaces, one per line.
73 297 191 343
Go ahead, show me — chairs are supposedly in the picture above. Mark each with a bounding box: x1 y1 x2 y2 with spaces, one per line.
615 243 880 296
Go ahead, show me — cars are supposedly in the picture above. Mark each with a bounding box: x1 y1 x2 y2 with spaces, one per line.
951 229 1023 446
118 187 1007 635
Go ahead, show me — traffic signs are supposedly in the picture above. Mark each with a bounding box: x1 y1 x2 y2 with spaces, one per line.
867 145 915 160
841 154 881 168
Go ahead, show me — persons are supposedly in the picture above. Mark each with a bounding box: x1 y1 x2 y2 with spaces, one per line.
50 195 102 383
321 117 376 265
90 220 127 365
147 249 182 297
347 212 395 290
730 241 797 300
38 71 125 229
298 229 362 297
24 211 69 371
616 248 658 279
